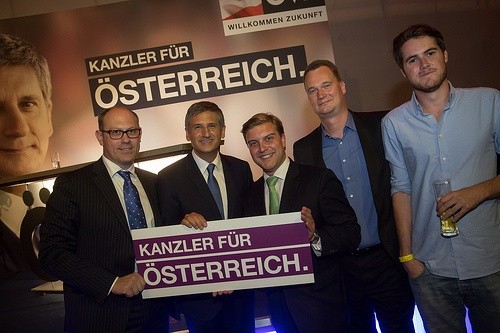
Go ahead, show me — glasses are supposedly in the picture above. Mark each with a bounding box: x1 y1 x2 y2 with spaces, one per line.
99 127 143 140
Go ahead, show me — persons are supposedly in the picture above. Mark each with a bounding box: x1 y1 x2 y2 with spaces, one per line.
381 24 500 333
0 34 53 280
293 60 416 333
39 107 170 333
244 113 361 333
157 101 254 333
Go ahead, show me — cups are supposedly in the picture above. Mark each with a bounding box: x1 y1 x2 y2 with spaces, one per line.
50 152 61 169
432 179 459 236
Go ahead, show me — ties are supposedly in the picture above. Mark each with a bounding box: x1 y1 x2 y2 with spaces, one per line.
265 176 281 215
206 163 225 222
117 170 148 229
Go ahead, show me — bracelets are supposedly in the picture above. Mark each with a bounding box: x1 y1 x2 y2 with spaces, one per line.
399 254 414 262
311 231 319 244
